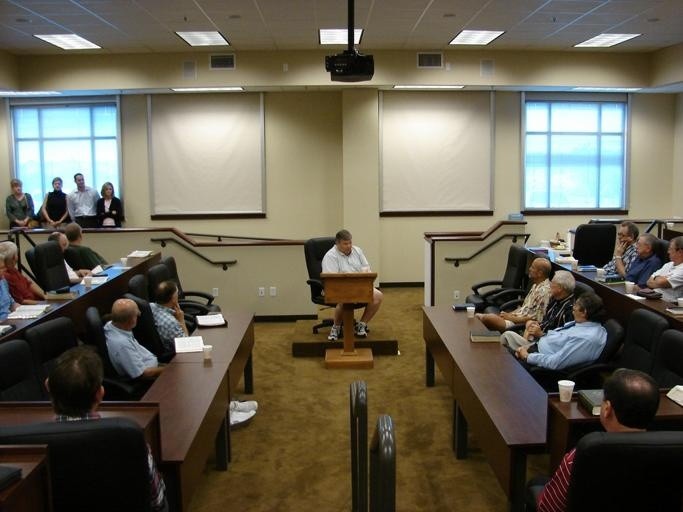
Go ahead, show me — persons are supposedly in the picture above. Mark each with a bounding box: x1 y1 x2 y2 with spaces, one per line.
0 221 112 327
96 181 122 229
472 218 683 373
101 298 258 427
6 178 35 230
147 281 188 354
66 172 101 230
43 344 172 511
41 176 70 230
320 228 384 343
536 368 659 512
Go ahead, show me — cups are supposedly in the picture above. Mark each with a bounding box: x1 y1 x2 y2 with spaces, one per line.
202 344 213 360
120 257 127 267
666 222 674 230
571 259 577 269
556 379 575 403
466 306 474 318
624 281 635 293
83 277 92 287
677 298 682 307
596 268 604 277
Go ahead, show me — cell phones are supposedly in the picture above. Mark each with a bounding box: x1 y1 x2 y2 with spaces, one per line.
559 252 571 257
640 288 655 293
92 274 108 277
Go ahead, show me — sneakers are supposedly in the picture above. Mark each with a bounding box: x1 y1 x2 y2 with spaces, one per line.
229 400 258 412
230 410 256 425
328 325 341 341
357 322 367 336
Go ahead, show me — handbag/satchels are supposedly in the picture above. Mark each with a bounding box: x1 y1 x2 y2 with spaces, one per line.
37 202 48 222
28 220 39 228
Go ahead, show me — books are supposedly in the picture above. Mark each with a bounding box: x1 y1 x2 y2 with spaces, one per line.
196 314 226 327
0 323 15 337
174 336 203 354
0 465 22 489
666 385 682 408
452 302 476 310
469 329 501 343
578 388 606 417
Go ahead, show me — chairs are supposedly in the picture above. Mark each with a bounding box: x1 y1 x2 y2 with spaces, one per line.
303 236 363 335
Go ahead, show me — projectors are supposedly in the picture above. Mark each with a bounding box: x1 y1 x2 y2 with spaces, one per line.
325 55 374 81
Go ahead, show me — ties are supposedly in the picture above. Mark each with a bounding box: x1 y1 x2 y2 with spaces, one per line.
535 322 576 344
